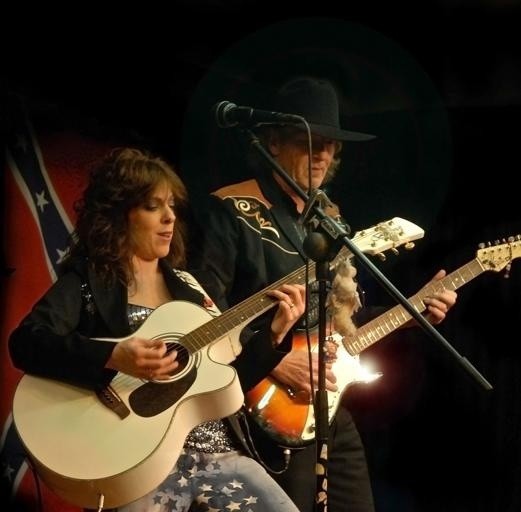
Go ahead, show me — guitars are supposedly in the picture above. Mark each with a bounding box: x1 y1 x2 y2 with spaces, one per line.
13 216 425 509
244 235 521 448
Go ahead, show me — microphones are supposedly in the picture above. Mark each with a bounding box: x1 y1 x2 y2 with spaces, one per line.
211 100 305 129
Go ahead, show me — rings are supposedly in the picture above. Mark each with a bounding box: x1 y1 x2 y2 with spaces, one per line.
144 370 152 377
290 303 295 309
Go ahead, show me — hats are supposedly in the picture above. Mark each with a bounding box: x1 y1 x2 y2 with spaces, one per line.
265 76 376 141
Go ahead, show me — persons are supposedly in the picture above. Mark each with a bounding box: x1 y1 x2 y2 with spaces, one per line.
8 146 309 511
195 73 459 511
